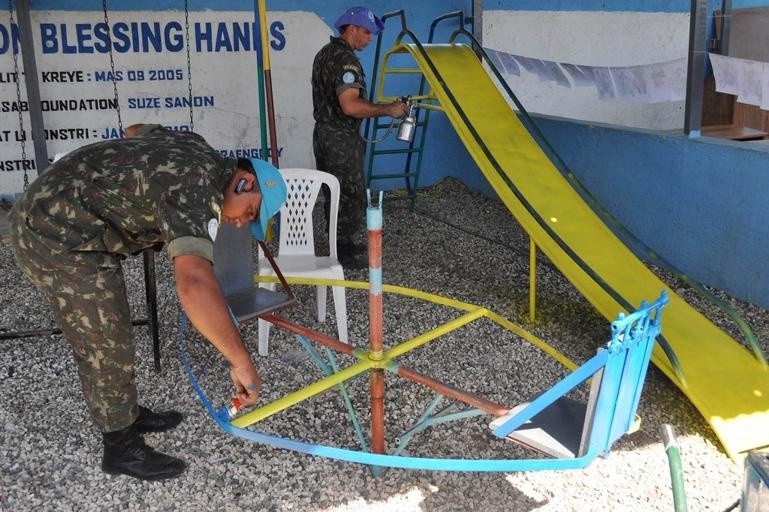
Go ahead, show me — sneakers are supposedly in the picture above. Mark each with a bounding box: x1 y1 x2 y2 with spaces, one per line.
330 236 371 271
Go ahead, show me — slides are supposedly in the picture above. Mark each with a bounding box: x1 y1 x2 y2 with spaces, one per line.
406 42 769 461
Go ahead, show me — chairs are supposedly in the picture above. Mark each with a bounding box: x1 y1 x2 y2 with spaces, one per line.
254 165 351 357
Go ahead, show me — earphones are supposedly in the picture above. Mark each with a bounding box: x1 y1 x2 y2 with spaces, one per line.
234 177 247 194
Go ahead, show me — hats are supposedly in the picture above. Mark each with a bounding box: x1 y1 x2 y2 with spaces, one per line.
338 6 386 41
244 155 288 244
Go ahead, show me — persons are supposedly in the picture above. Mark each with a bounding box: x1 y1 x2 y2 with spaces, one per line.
7 123 287 480
311 7 410 270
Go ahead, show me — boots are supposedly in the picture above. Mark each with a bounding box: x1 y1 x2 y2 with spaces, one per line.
97 404 185 480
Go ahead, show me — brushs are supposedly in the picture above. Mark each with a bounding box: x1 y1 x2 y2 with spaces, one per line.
220 392 249 422
